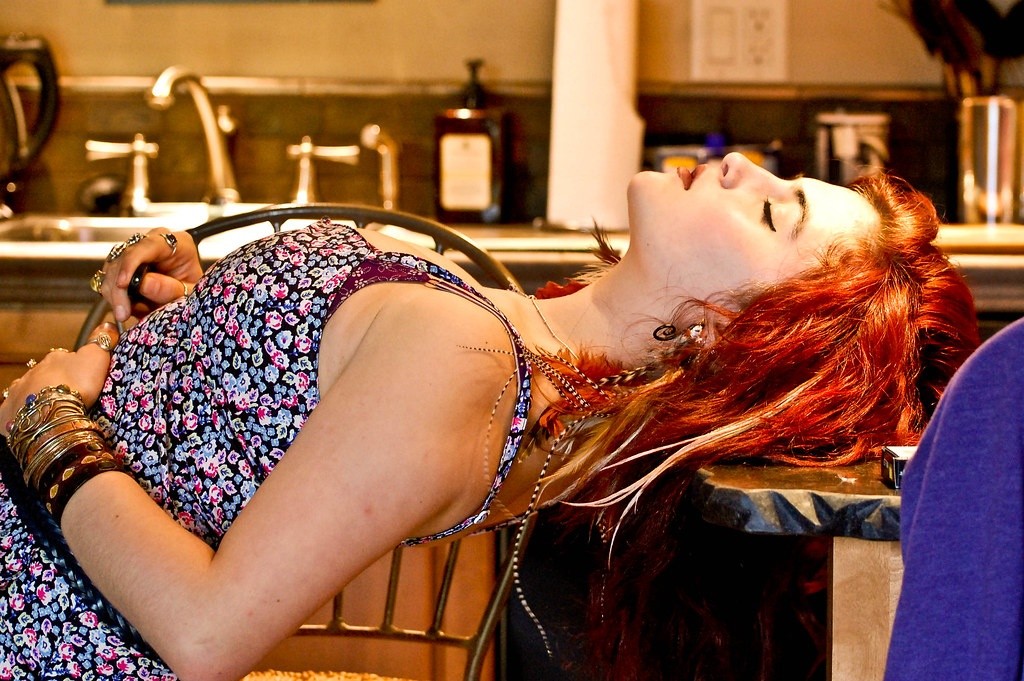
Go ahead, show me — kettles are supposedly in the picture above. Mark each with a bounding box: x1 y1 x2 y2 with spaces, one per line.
0 36 61 216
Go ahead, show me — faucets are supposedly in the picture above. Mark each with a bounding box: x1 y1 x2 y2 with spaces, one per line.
147 63 241 204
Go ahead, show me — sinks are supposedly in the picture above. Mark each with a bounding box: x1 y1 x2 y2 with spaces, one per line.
0 211 197 259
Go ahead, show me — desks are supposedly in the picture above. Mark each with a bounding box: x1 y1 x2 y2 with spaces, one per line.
490 455 902 681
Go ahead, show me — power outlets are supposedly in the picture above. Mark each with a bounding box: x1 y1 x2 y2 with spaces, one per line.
691 2 789 85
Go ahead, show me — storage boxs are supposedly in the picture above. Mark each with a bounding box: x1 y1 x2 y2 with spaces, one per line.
881 445 922 489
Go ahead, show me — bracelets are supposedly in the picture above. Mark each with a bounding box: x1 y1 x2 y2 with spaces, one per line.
6 384 134 529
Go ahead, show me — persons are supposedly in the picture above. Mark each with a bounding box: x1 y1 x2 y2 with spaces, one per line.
0 151 977 681
884 311 1024 681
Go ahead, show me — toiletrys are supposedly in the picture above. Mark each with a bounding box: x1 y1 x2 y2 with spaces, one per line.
430 54 516 227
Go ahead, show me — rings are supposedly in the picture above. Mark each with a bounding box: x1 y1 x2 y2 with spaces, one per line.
159 233 177 257
105 243 126 262
1 388 9 398
50 347 69 353
180 281 188 296
89 270 106 293
125 233 141 246
88 333 114 356
27 359 37 369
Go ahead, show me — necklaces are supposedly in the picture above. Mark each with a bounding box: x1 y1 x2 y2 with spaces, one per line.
508 282 579 361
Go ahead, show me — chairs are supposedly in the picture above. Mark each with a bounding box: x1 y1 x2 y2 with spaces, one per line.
75 203 536 681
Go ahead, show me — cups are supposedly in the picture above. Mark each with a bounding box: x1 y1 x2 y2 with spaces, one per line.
816 112 891 186
959 97 1017 224
